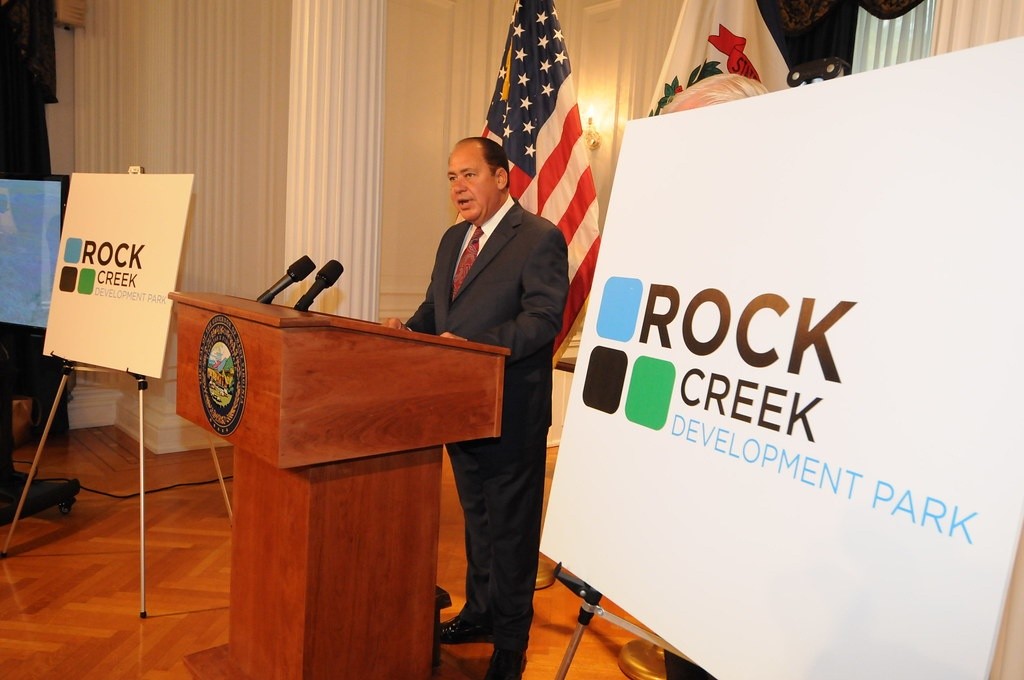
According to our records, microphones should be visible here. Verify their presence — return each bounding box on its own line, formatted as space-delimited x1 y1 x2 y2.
258 255 316 304
293 260 344 311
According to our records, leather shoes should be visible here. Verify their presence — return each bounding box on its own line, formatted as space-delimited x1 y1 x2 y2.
485 647 527 680
440 616 496 643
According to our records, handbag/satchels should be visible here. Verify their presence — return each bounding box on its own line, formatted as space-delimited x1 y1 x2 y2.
12 395 43 447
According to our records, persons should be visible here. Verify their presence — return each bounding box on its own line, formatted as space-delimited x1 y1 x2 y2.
378 136 570 680
661 73 769 679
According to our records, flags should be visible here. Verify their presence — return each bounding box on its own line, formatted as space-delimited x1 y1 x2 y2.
646 1 806 117
455 1 602 366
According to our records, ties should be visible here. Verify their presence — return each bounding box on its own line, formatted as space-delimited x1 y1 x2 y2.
450 226 484 302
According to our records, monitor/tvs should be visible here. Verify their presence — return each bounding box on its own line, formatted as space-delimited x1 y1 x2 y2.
0 170 70 336
